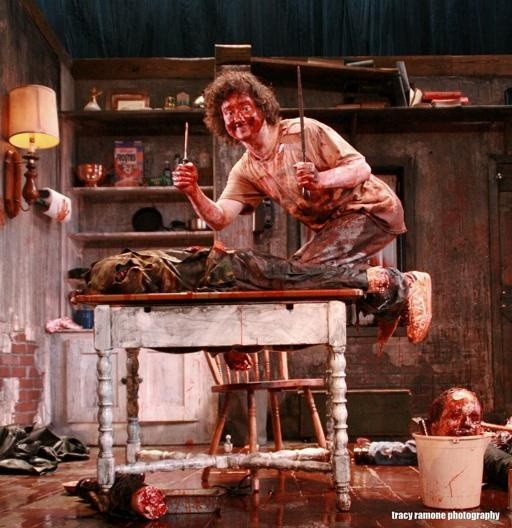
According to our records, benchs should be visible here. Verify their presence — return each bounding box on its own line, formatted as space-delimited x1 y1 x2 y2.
293 387 413 442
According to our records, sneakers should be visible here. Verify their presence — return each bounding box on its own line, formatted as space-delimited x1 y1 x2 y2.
400 269 433 345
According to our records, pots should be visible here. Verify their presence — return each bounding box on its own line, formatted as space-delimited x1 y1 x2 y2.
131 205 175 233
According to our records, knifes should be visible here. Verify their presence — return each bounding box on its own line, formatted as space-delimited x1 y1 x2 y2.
296 64 311 199
182 122 190 164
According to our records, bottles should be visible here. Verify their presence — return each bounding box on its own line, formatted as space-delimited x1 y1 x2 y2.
161 160 172 179
173 153 181 169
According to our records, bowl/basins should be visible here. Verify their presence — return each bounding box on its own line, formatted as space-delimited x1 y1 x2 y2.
189 216 211 230
62 479 81 496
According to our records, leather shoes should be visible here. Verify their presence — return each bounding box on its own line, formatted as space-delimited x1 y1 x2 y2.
353 436 370 465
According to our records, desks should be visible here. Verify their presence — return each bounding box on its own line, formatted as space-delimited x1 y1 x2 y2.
70 288 364 512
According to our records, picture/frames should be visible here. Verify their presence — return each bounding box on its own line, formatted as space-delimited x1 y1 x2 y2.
295 164 409 330
104 85 149 112
114 98 151 111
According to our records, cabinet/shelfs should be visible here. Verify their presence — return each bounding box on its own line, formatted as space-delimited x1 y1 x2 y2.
63 44 512 294
54 330 221 446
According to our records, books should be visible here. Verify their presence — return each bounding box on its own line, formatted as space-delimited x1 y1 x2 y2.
410 87 469 109
213 43 252 66
307 56 344 67
336 103 360 109
345 58 375 66
359 101 386 108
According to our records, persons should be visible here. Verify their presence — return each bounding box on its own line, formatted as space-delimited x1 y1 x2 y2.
427 387 483 436
68 248 434 374
172 68 410 358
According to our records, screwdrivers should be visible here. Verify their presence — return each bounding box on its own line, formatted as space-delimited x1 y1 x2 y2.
183 122 189 165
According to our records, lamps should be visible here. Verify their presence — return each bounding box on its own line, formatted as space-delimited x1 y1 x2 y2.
2 86 61 219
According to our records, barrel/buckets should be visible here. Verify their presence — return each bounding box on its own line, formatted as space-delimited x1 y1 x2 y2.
411 429 496 510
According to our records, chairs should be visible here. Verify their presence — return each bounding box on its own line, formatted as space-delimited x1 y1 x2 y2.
202 348 331 493
